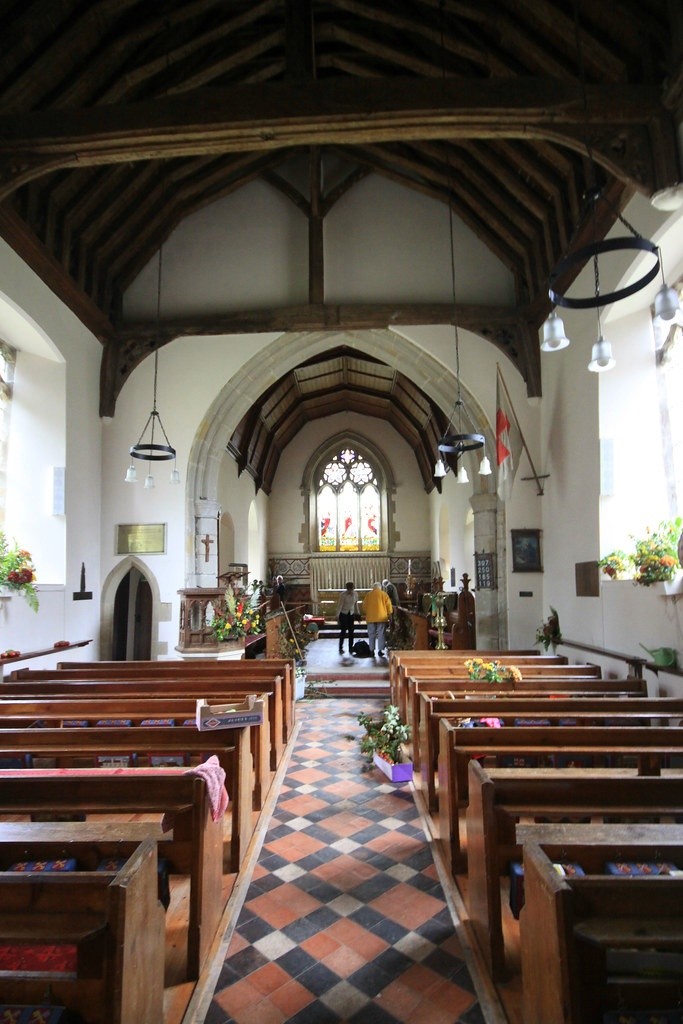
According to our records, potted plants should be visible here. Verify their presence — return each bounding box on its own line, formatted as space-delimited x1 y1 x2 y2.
353 700 416 783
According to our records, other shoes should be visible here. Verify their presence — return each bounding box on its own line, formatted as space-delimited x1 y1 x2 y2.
339 650 344 655
349 647 354 653
378 652 384 656
370 652 375 657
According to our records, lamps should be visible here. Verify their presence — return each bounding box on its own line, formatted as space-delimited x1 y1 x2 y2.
533 1 683 381
123 212 187 489
433 162 496 486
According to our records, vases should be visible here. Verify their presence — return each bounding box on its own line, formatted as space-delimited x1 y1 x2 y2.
227 636 247 647
602 567 621 583
292 675 308 702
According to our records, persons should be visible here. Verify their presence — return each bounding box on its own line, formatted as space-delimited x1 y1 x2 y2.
275 575 285 606
336 581 361 654
362 584 393 656
382 580 400 635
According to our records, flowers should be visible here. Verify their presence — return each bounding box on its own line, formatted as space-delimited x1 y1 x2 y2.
204 582 263 642
269 615 307 670
0 531 44 615
595 551 630 575
627 517 682 587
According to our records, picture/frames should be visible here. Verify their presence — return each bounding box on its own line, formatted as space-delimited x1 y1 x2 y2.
510 525 545 579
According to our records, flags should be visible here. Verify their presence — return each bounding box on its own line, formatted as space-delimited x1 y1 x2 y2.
495 368 525 501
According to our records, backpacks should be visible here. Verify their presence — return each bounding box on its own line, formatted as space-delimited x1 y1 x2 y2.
350 641 371 658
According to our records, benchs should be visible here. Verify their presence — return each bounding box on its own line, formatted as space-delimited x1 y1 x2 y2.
386 646 683 1024
0 654 301 1024
